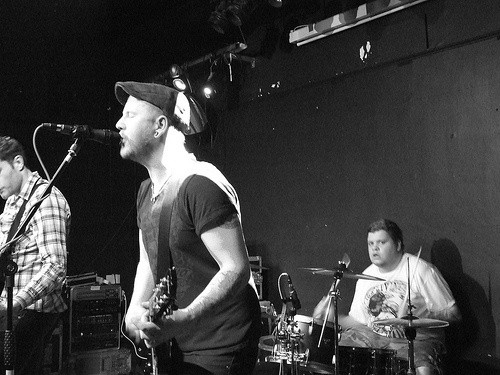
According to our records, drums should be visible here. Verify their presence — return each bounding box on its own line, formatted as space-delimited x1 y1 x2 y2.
269 313 343 365
334 345 398 375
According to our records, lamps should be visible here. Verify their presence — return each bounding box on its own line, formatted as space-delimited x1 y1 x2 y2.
289 0 430 46
168 0 282 99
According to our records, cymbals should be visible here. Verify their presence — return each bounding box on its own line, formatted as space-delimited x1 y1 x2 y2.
373 317 450 329
249 264 270 270
295 265 387 281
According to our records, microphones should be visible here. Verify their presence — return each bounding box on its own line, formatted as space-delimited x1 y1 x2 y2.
287 275 302 309
41 122 122 146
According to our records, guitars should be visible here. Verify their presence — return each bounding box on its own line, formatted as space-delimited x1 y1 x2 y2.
130 266 178 374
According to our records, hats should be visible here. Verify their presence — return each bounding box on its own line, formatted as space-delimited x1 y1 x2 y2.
115 81 191 136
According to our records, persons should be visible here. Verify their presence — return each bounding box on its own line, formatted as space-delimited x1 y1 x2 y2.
314 219 461 375
0 136 71 375
114 81 262 375
